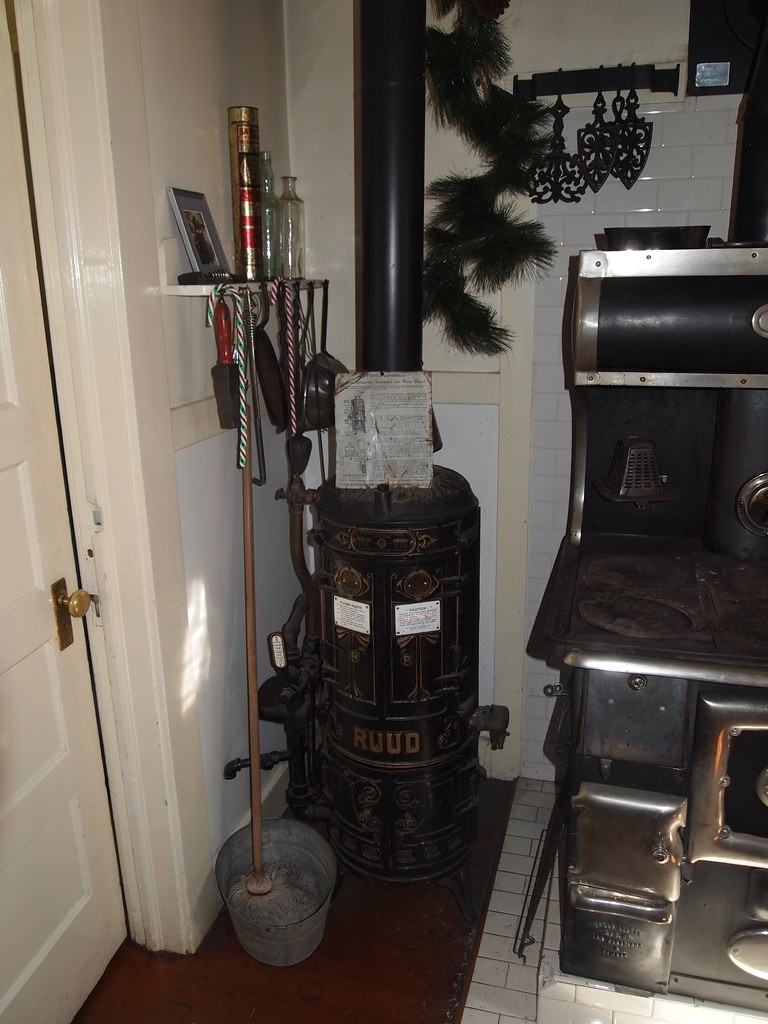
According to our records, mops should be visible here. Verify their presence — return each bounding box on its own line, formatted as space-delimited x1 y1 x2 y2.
226 400 321 929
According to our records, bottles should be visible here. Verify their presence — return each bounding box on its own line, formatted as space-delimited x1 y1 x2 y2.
279 175 305 279
259 151 283 279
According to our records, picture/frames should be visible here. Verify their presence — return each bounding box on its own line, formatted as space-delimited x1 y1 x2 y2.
167 186 232 280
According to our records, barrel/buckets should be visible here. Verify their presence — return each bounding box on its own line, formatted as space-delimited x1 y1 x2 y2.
215 818 345 966
